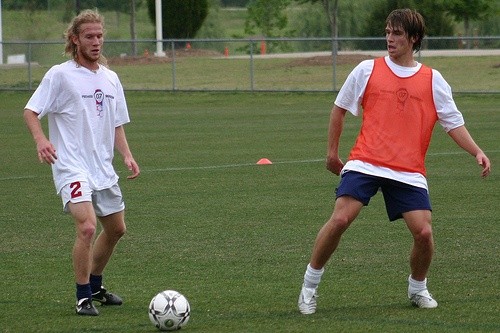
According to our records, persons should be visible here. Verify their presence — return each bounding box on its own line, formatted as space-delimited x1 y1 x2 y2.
298 6 492 316
23 7 142 315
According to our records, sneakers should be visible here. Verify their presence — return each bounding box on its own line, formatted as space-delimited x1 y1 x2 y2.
75 297 99 316
92 286 123 305
298 283 319 315
408 286 438 309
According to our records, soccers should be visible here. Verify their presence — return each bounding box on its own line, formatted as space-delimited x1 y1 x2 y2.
148 289 190 331
